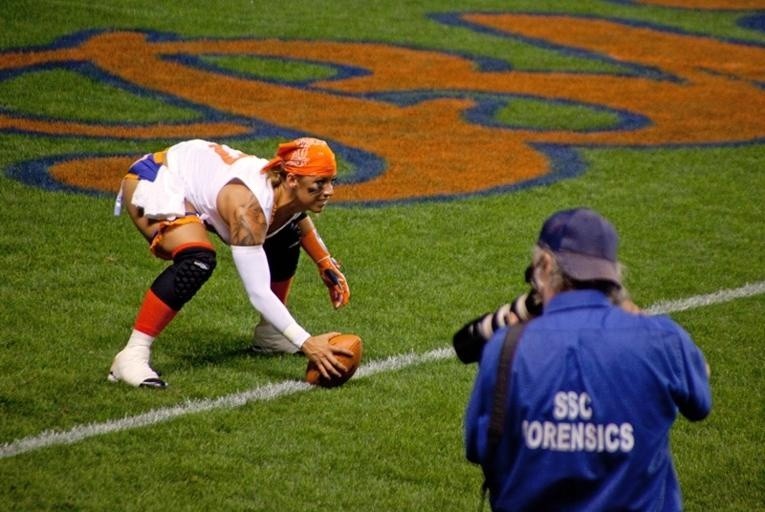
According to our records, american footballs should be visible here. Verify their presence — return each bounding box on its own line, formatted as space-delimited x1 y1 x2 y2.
306 335 362 387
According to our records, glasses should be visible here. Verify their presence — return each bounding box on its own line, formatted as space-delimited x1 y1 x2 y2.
522 253 548 286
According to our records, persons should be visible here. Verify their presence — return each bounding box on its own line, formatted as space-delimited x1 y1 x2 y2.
462 205 713 512
106 134 354 390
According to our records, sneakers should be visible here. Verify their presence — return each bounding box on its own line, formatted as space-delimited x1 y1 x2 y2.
106 343 169 390
250 321 306 357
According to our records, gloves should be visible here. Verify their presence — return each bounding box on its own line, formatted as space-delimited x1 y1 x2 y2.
318 258 350 309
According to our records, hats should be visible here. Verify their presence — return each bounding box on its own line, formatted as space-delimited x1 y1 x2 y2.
537 205 623 290
